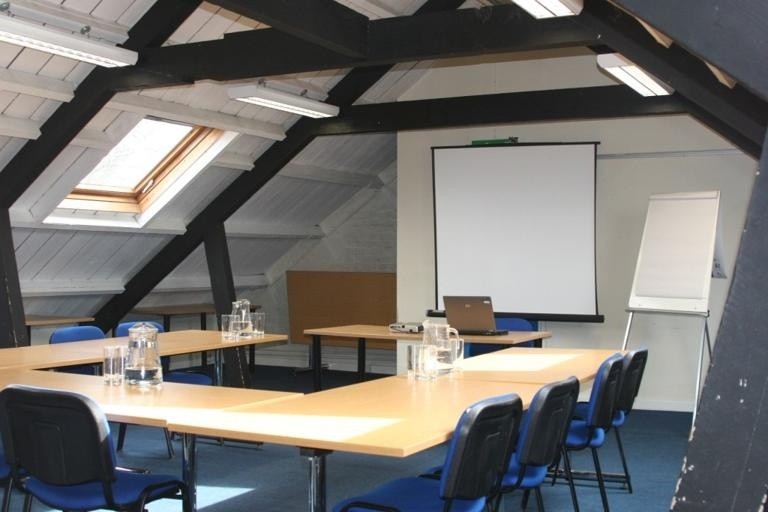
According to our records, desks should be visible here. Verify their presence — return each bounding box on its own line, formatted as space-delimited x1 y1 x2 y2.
0 329 289 445
302 324 552 391
0 368 305 512
131 303 262 374
24 314 95 346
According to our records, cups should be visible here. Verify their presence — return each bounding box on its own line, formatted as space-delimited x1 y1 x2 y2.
255 313 264 335
222 314 241 340
412 343 438 381
102 346 122 385
452 338 465 369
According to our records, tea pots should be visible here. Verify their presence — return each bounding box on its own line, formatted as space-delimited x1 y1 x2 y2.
423 320 460 375
124 320 164 394
231 298 253 339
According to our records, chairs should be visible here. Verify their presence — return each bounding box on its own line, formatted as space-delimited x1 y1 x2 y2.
421 375 579 512
330 393 523 510
565 352 625 512
115 322 212 458
49 326 107 375
1 383 187 511
552 346 648 494
462 316 539 358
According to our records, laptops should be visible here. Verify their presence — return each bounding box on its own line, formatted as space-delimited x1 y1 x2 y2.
443 296 508 336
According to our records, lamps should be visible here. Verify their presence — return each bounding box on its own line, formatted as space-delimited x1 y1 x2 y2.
226 78 341 122
1 3 139 70
595 53 673 100
512 0 585 21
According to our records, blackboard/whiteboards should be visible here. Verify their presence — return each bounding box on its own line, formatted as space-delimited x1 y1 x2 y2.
625 190 721 317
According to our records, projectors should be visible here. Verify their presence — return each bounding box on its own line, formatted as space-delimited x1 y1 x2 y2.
389 322 423 333
